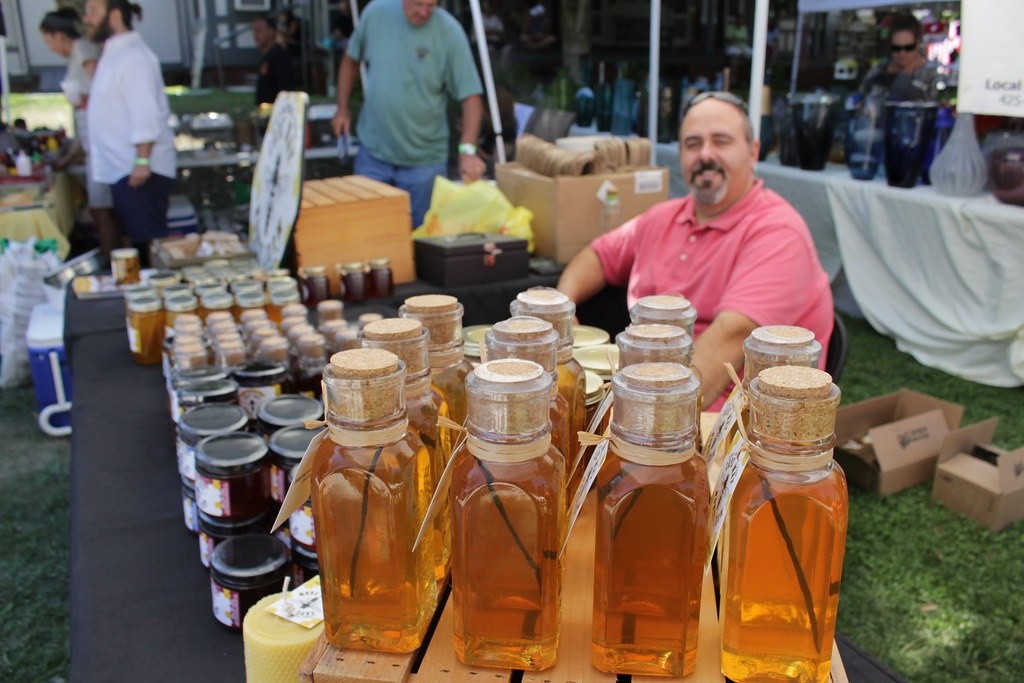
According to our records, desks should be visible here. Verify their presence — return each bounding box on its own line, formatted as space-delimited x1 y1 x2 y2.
63 266 912 683
0 103 349 262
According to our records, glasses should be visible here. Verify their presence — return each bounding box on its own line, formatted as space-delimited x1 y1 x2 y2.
890 37 918 51
684 91 748 116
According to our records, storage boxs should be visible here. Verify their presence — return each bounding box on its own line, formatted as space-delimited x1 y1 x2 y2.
167 188 199 238
28 302 73 437
833 389 966 500
932 416 1023 533
293 175 417 282
494 159 669 265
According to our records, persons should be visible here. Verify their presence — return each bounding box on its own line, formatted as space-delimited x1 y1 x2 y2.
557 92 834 413
332 0 488 229
84 0 184 267
40 7 102 140
858 12 939 101
253 17 293 135
276 0 354 54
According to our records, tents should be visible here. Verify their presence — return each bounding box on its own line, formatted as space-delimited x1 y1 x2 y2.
790 0 930 96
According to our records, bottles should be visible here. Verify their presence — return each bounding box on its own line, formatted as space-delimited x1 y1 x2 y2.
596 56 726 144
111 248 849 678
6 134 59 185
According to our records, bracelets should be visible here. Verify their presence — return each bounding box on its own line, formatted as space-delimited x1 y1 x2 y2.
135 158 150 166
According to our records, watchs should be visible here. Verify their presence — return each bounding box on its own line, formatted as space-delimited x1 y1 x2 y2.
458 143 477 155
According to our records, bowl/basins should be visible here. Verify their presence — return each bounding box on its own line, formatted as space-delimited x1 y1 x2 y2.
43 247 102 289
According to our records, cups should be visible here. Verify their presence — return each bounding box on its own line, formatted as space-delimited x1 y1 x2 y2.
884 98 939 188
790 92 841 171
